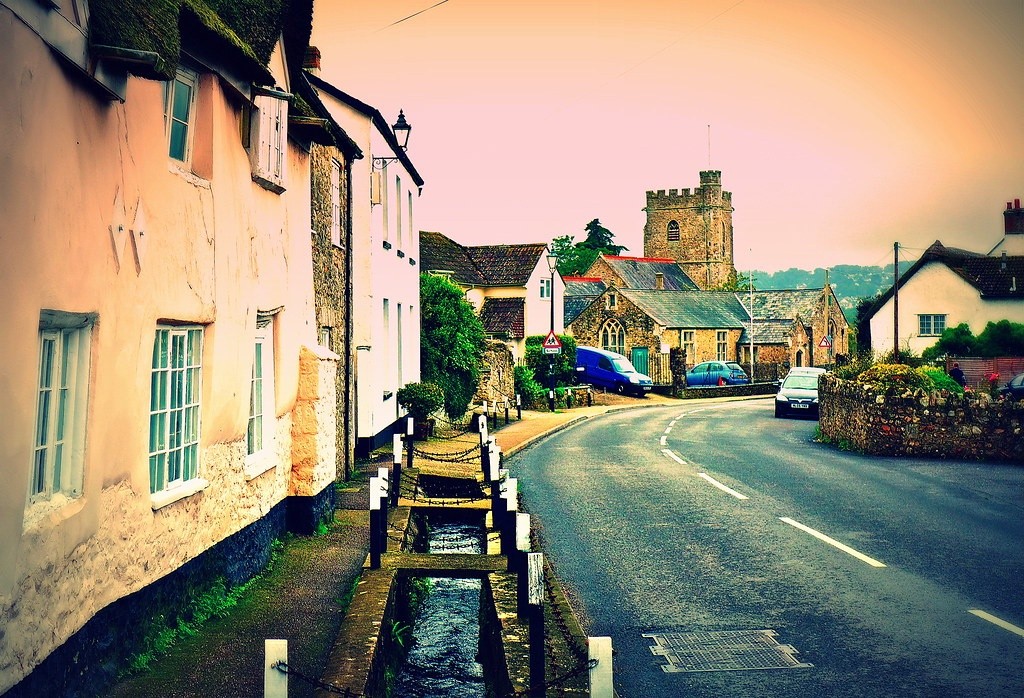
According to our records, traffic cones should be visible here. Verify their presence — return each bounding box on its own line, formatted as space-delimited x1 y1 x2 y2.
718 376 723 386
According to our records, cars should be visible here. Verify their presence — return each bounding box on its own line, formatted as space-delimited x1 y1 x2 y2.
686 360 750 386
775 366 827 418
997 373 1023 400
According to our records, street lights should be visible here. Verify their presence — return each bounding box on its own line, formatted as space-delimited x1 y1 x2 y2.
546 246 559 411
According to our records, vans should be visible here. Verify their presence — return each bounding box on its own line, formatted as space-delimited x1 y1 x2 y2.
576 346 652 395
